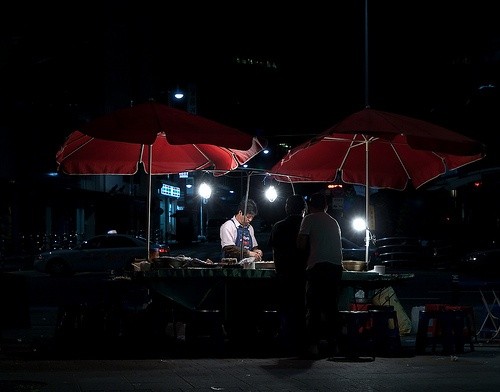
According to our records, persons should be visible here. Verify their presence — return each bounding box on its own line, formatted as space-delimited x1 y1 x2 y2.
269 195 310 356
220 199 263 262
297 200 341 359
168 208 198 248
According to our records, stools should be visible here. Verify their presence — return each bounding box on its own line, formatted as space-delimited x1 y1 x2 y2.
338 304 476 358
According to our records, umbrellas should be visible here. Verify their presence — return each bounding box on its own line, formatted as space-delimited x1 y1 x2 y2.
56 98 265 262
266 106 489 265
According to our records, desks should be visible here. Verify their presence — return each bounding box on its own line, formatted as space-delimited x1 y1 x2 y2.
121 267 416 359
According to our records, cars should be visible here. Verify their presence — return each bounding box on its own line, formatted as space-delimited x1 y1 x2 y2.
34 229 170 273
342 232 376 263
